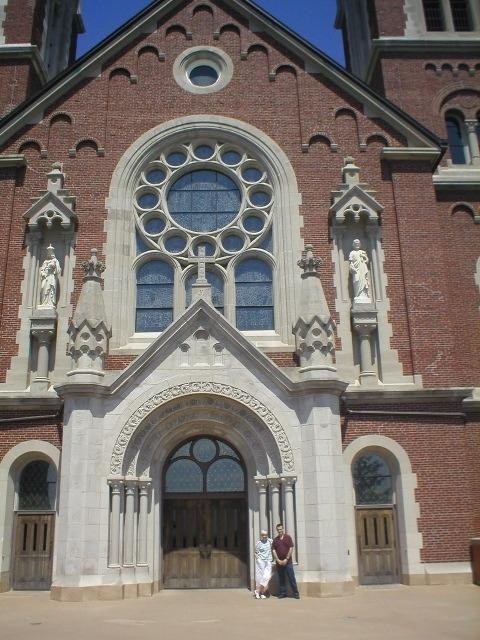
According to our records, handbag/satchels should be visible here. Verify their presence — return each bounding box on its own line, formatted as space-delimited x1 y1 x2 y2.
285 557 290 560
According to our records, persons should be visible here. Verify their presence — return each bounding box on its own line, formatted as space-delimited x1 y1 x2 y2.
349 239 370 297
254 530 276 599
272 524 299 599
41 247 62 305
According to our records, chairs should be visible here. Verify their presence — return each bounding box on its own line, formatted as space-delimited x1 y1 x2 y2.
253 589 271 599
279 593 288 598
293 592 300 599
